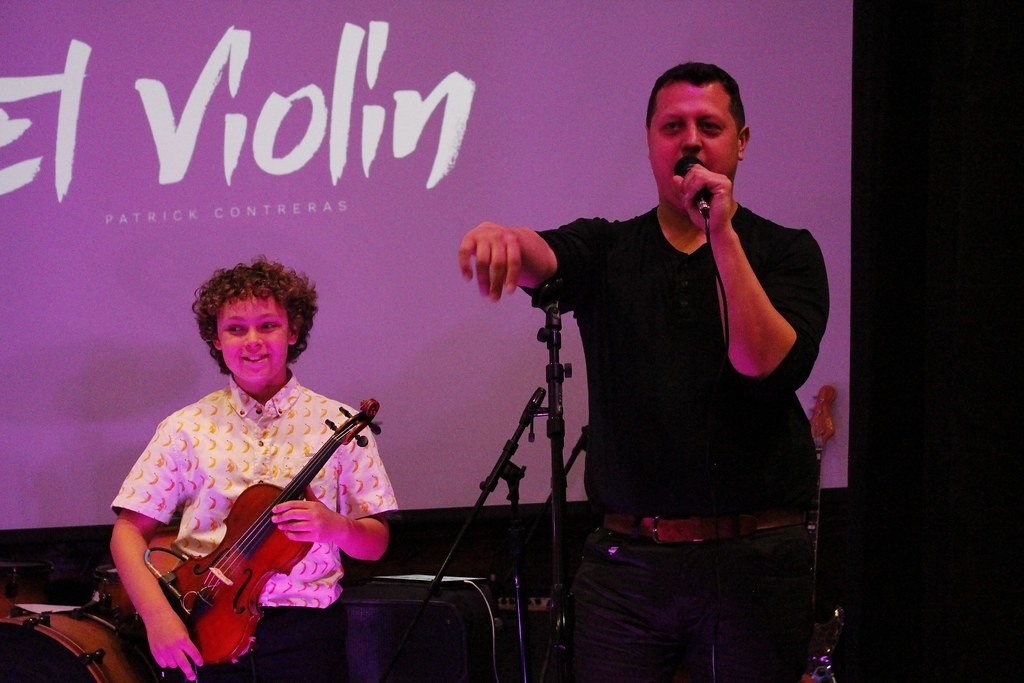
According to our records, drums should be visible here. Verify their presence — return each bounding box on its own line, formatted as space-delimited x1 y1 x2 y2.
0 603 160 683
0 560 55 617
91 560 143 625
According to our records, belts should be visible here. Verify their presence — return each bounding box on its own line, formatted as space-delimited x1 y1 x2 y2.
604 507 807 545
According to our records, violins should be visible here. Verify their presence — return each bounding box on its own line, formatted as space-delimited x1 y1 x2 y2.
142 397 383 664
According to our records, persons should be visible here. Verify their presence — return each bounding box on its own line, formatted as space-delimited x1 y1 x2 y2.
109 254 399 683
459 61 830 683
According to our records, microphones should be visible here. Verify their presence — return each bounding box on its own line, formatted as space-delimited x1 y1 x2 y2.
675 155 709 219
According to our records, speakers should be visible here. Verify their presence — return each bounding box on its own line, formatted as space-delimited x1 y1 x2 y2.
344 583 495 683
496 596 575 683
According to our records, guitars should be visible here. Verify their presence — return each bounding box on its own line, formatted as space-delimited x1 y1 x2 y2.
797 383 845 683
